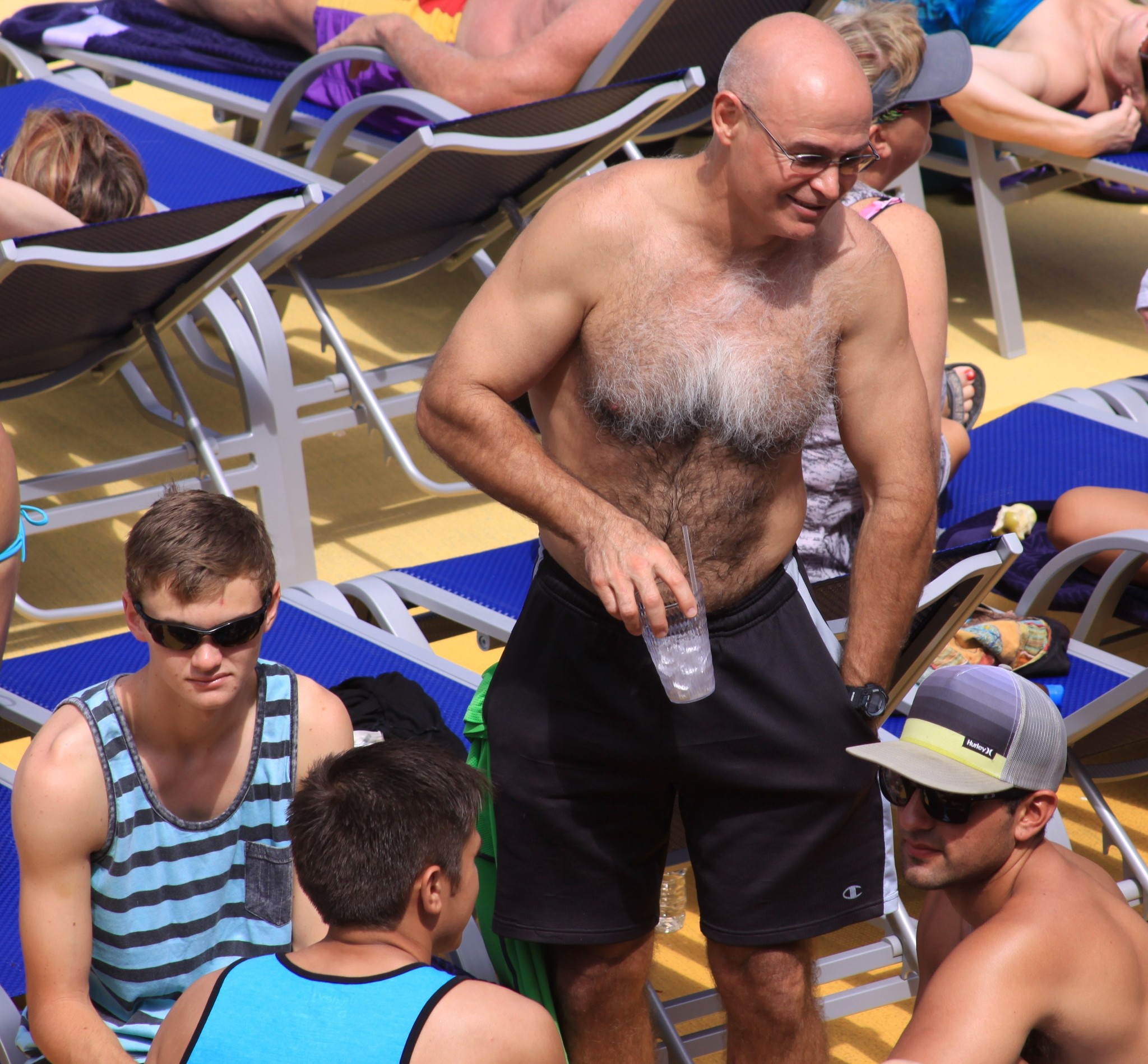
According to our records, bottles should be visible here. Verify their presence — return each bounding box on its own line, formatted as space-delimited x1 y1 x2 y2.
654 868 687 935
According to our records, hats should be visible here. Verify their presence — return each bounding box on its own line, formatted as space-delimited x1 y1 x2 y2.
870 29 973 119
845 664 1067 795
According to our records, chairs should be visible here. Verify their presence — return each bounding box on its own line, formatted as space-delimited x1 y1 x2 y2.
0 0 1148 1064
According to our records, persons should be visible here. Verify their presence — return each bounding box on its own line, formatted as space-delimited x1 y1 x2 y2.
0 0 1148 1064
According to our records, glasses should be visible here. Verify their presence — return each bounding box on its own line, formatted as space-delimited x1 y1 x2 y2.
879 764 1033 824
132 590 273 650
0 146 12 176
875 98 931 123
739 100 880 175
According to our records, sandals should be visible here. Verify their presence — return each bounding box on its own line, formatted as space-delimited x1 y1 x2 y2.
941 363 986 433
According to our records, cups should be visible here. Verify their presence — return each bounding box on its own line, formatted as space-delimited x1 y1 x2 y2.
635 577 716 705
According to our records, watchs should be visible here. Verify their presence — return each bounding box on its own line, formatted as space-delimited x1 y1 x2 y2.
845 683 889 719
